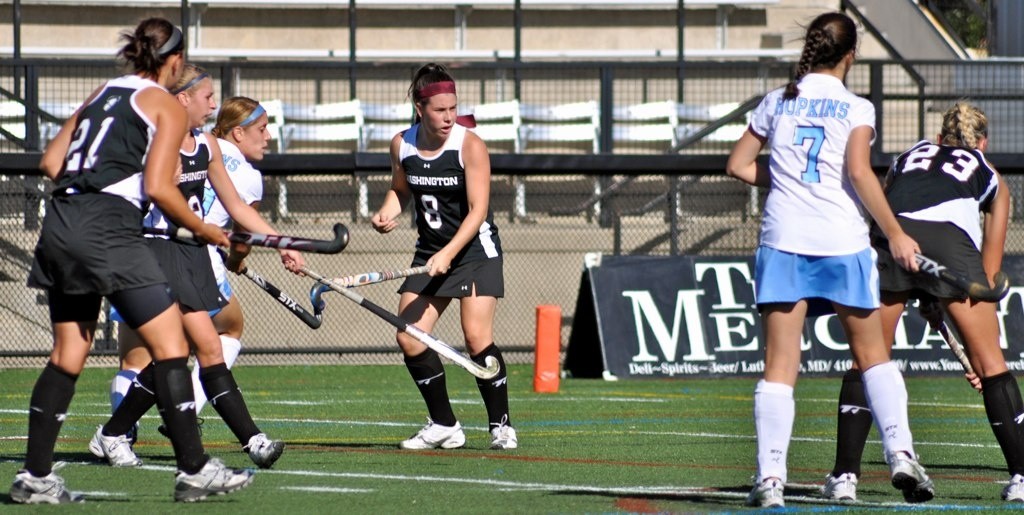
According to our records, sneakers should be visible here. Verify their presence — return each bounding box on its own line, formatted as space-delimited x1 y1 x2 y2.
489 413 518 449
159 418 205 438
888 451 935 503
819 472 858 500
400 417 464 449
243 433 284 469
174 457 254 501
88 423 137 466
746 478 785 508
10 461 84 504
1002 474 1024 501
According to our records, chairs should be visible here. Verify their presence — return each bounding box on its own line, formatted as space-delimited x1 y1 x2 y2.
0 99 763 224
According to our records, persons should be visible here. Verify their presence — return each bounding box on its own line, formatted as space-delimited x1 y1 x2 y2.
371 61 518 451
725 11 935 508
86 63 309 469
823 104 1024 502
7 18 254 506
109 96 272 447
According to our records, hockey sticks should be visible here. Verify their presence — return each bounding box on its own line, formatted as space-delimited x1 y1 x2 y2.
920 304 982 383
220 251 322 330
309 267 443 311
133 221 351 256
289 261 500 379
867 231 1010 303
